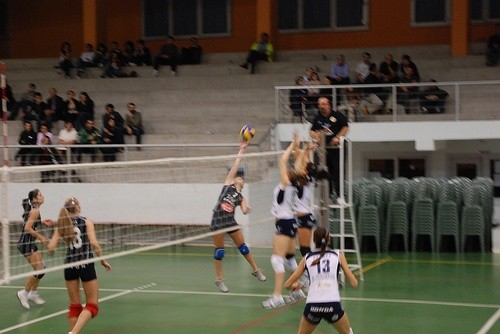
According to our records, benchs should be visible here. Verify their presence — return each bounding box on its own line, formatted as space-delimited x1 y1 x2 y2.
0 43 500 181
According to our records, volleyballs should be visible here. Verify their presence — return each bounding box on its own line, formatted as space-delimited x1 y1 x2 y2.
239 124 257 143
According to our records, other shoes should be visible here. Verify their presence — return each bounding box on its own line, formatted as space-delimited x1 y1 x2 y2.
319 197 335 204
336 197 346 205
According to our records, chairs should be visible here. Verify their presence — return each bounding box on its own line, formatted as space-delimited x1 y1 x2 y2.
326 175 495 255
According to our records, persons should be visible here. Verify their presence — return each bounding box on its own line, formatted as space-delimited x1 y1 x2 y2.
16 189 49 310
54 35 204 79
283 226 358 334
309 96 348 206
210 141 267 292
0 79 144 183
262 131 330 310
240 32 274 75
47 197 112 334
289 52 452 115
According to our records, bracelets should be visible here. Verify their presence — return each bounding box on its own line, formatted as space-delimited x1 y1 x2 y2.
335 135 340 140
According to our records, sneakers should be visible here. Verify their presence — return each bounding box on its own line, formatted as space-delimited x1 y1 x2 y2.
28 291 46 305
215 279 228 292
286 289 307 305
252 268 267 281
17 289 30 309
299 277 310 288
261 296 287 309
